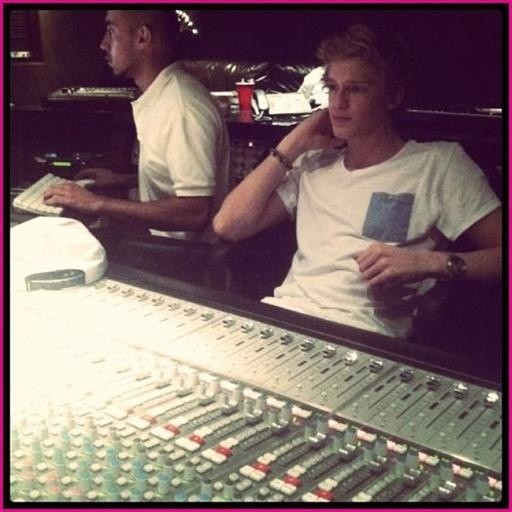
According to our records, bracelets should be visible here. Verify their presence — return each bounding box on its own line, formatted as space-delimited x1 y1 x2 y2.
270 148 294 171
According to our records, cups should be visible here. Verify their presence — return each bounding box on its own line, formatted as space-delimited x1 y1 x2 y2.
235 81 255 111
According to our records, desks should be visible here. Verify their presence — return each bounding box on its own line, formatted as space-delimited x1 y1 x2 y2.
7 94 316 299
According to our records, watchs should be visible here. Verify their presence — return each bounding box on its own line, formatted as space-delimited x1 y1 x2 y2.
445 251 469 285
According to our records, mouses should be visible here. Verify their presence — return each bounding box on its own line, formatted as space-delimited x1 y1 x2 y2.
76 178 96 188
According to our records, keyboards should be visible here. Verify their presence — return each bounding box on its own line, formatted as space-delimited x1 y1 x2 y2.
12 171 85 217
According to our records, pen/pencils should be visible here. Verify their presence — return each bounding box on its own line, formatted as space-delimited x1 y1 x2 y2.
51 161 74 167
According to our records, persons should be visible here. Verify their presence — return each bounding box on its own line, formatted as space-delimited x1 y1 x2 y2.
212 25 502 340
43 8 232 293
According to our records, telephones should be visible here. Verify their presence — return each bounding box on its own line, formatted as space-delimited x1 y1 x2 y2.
250 89 313 116
